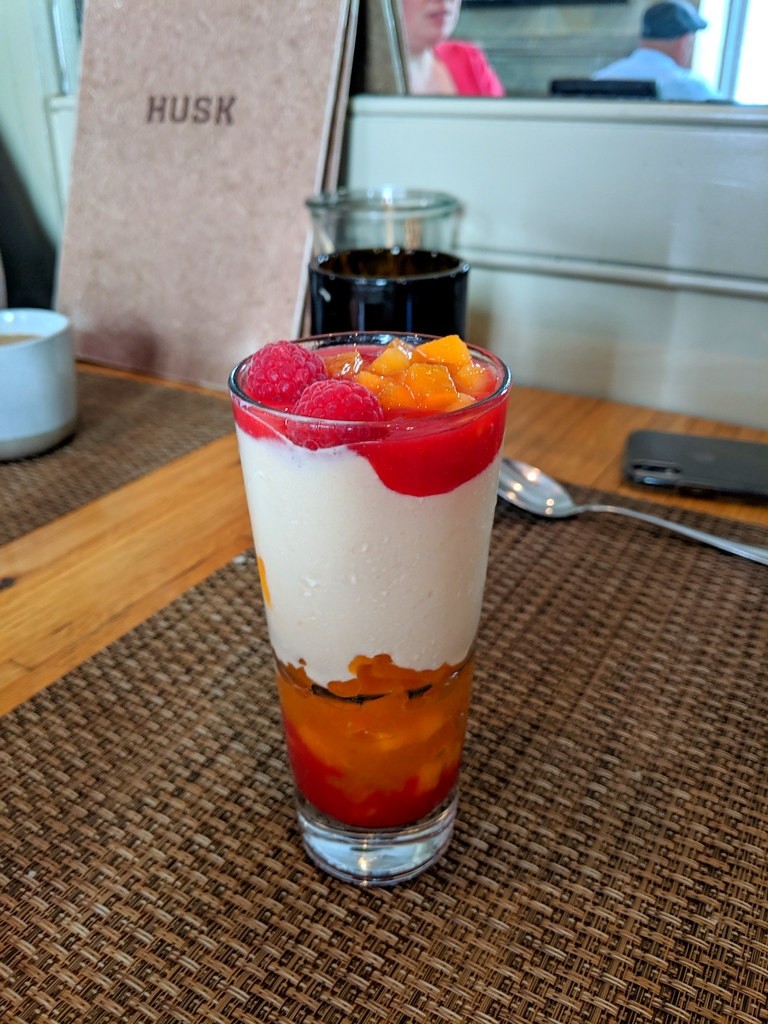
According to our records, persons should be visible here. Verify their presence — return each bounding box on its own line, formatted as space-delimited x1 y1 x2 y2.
592 0 724 102
398 0 504 97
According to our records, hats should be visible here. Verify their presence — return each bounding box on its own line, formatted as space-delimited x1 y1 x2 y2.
641 2 708 38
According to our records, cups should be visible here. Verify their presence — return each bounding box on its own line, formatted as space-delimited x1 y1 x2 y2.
226 331 515 887
305 185 473 343
0 309 81 460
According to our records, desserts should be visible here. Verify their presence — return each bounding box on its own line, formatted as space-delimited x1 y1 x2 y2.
231 335 510 830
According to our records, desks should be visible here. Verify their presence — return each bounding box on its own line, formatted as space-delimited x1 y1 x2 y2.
0 359 768 1024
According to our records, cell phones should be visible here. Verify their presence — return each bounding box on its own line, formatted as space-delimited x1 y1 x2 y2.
620 428 768 505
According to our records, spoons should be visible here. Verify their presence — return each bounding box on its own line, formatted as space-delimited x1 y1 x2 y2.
497 456 767 566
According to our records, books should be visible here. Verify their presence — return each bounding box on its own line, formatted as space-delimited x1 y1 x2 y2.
49 0 357 394
